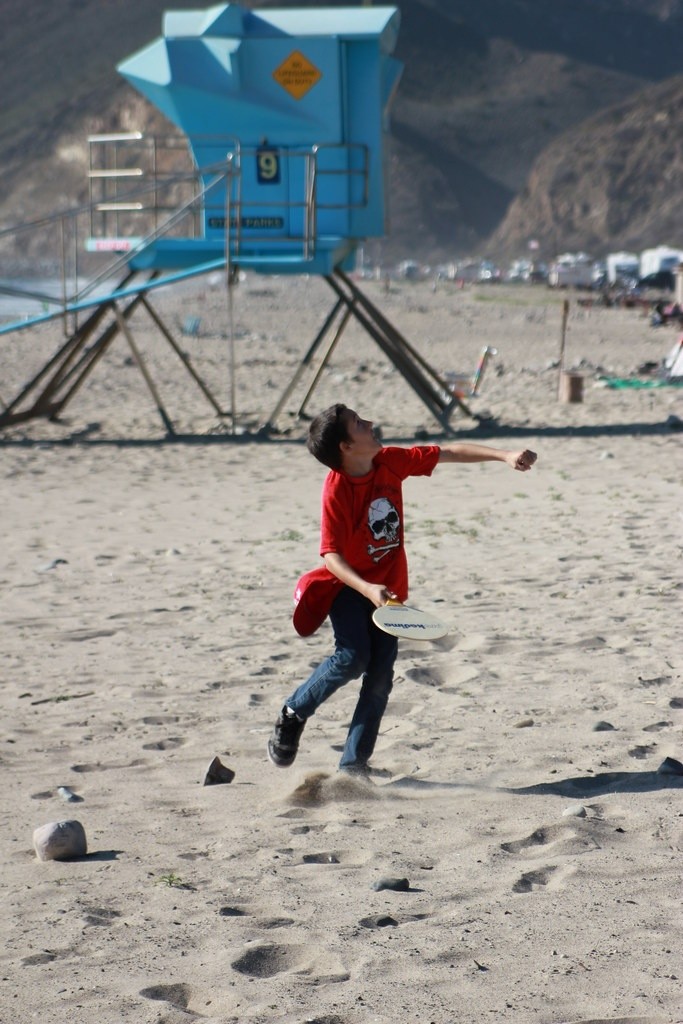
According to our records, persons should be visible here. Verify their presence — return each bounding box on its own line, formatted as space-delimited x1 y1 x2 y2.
269 404 538 784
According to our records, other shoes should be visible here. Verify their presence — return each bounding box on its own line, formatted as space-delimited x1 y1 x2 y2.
338 769 376 786
268 705 307 769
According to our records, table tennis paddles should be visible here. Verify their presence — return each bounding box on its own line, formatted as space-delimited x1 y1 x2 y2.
373 597 449 641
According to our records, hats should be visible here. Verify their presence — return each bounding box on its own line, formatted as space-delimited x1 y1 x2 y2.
292 567 344 638
